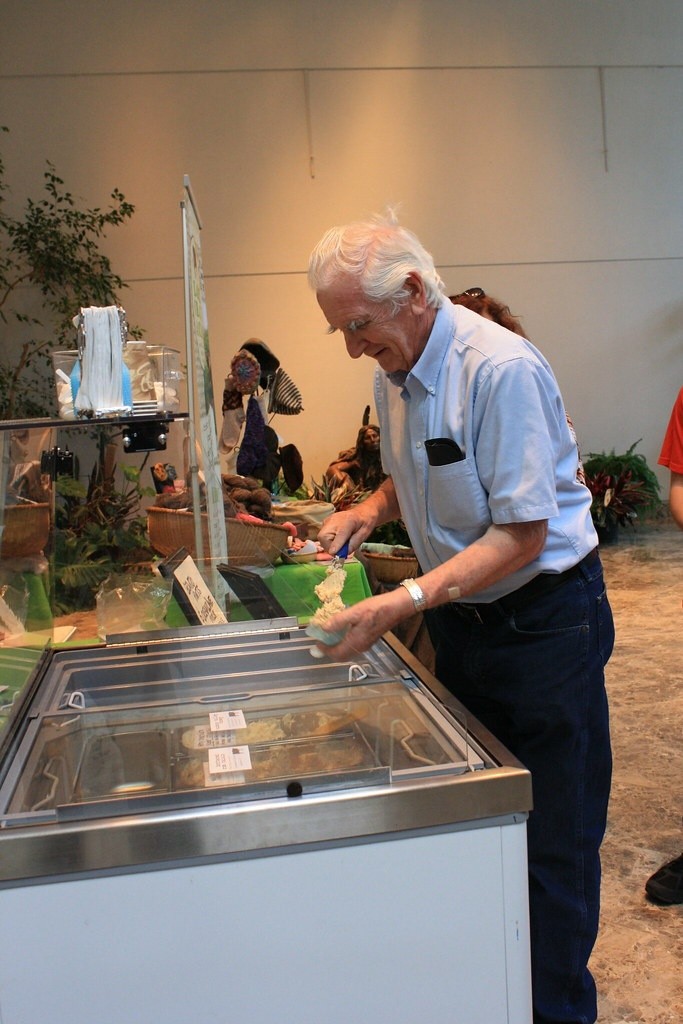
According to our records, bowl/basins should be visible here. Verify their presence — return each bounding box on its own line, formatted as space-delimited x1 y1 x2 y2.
305 622 349 644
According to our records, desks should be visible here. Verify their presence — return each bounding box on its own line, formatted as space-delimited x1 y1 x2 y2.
142 556 374 630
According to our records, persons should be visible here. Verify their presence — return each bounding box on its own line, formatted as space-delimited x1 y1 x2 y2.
310 207 618 1024
448 286 586 486
644 385 683 903
325 424 388 511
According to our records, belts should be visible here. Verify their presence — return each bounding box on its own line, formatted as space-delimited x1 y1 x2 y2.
444 544 599 624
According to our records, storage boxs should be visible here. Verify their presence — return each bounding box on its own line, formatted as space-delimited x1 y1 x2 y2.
48 344 181 418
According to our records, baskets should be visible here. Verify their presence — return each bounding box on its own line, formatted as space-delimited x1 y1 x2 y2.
146 505 291 567
361 550 418 583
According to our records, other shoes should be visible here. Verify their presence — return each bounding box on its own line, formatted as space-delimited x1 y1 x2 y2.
646 855 683 904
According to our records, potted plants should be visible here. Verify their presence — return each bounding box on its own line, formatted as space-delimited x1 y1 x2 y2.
581 466 657 536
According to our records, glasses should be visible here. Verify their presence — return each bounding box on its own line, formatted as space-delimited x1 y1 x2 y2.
448 286 484 302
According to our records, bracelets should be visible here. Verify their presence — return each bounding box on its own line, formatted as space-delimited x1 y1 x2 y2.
399 577 427 612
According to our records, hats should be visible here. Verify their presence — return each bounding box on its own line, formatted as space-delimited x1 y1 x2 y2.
267 369 302 416
230 350 261 394
238 338 280 389
279 444 303 494
